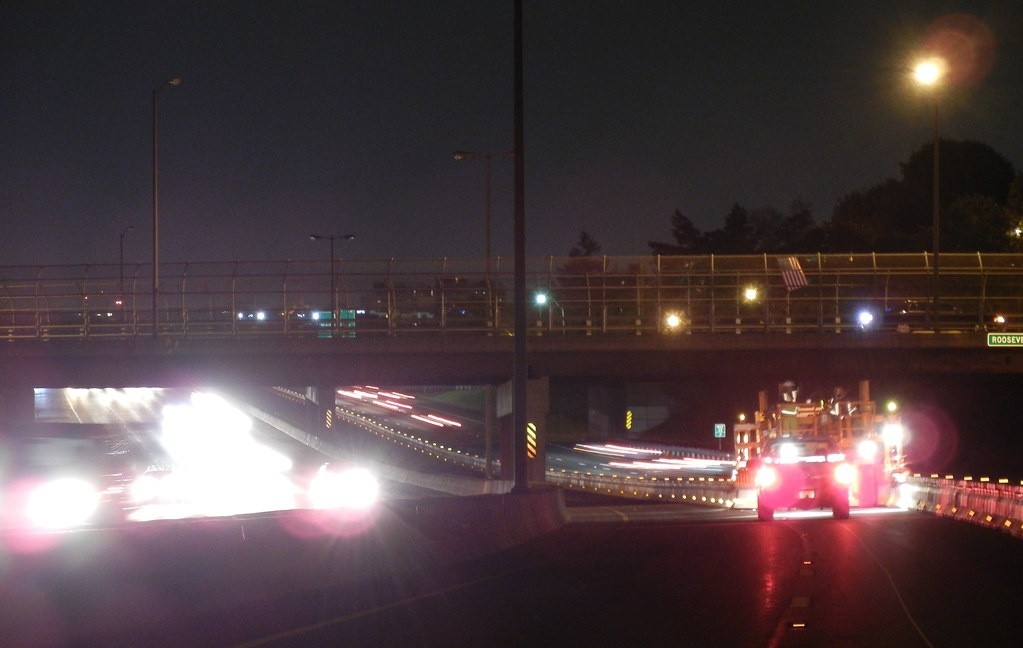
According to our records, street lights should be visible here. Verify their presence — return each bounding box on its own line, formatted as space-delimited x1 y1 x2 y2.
119 225 135 323
911 55 947 330
152 78 185 330
308 234 355 332
452 148 517 327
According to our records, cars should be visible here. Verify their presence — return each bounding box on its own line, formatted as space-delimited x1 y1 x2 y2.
756 435 853 521
